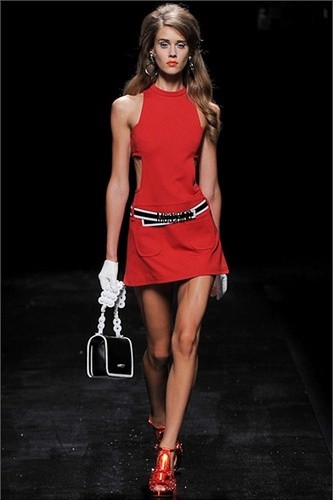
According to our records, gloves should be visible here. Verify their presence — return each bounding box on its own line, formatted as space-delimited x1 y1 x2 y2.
209 273 226 300
97 260 118 292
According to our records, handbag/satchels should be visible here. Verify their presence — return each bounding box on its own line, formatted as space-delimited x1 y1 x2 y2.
85 281 133 379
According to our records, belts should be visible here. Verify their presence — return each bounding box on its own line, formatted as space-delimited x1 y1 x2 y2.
130 199 208 227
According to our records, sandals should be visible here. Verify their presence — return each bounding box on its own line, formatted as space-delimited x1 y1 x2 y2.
149 442 178 496
147 414 182 457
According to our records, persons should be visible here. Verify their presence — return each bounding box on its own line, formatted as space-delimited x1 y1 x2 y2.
97 2 227 497
